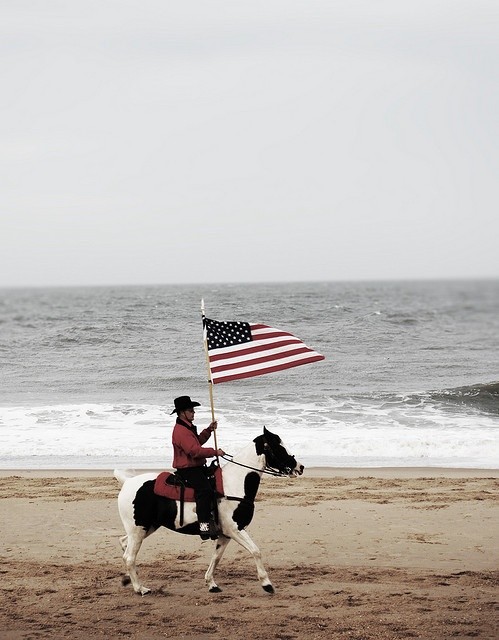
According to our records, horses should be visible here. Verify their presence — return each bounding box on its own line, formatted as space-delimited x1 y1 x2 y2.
113 426 305 596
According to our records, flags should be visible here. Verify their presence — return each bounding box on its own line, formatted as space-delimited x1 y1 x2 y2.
202 315 325 384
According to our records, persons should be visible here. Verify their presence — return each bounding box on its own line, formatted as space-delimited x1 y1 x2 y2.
170 396 224 540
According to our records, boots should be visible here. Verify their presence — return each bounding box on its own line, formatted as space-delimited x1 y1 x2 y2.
199 522 222 541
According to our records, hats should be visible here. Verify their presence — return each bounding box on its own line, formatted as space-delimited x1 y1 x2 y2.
170 395 202 423
